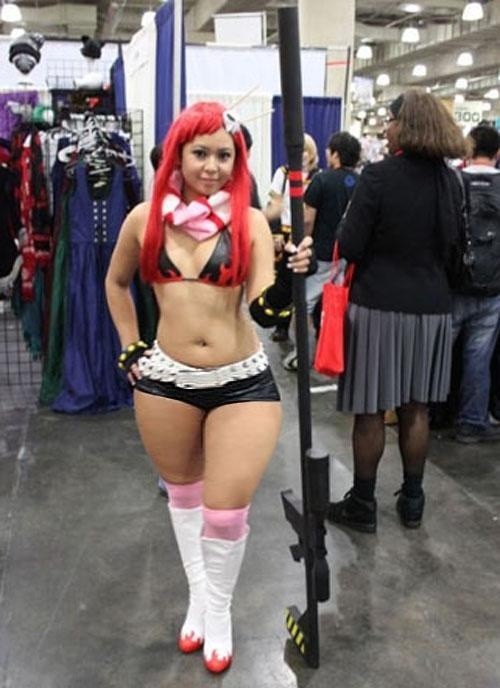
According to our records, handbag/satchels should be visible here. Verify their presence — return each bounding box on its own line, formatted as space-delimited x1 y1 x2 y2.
450 208 470 297
313 262 354 377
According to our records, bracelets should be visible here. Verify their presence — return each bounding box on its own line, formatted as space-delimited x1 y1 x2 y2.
118 338 149 376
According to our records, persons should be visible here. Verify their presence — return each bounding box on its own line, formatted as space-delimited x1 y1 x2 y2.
322 90 473 536
1 136 23 300
105 98 317 679
238 125 360 371
458 126 499 445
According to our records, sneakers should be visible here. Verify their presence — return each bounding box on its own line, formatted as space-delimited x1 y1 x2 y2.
269 326 298 371
396 492 425 528
455 425 500 444
326 495 377 532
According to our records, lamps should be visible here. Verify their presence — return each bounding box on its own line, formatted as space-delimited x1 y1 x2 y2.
356 1 499 111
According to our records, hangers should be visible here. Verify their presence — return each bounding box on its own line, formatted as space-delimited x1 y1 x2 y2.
59 117 135 168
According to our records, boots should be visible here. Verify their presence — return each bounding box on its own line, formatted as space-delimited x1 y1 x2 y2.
200 523 250 673
167 502 205 654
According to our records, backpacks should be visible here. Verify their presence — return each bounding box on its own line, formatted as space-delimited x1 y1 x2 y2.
468 176 500 298
